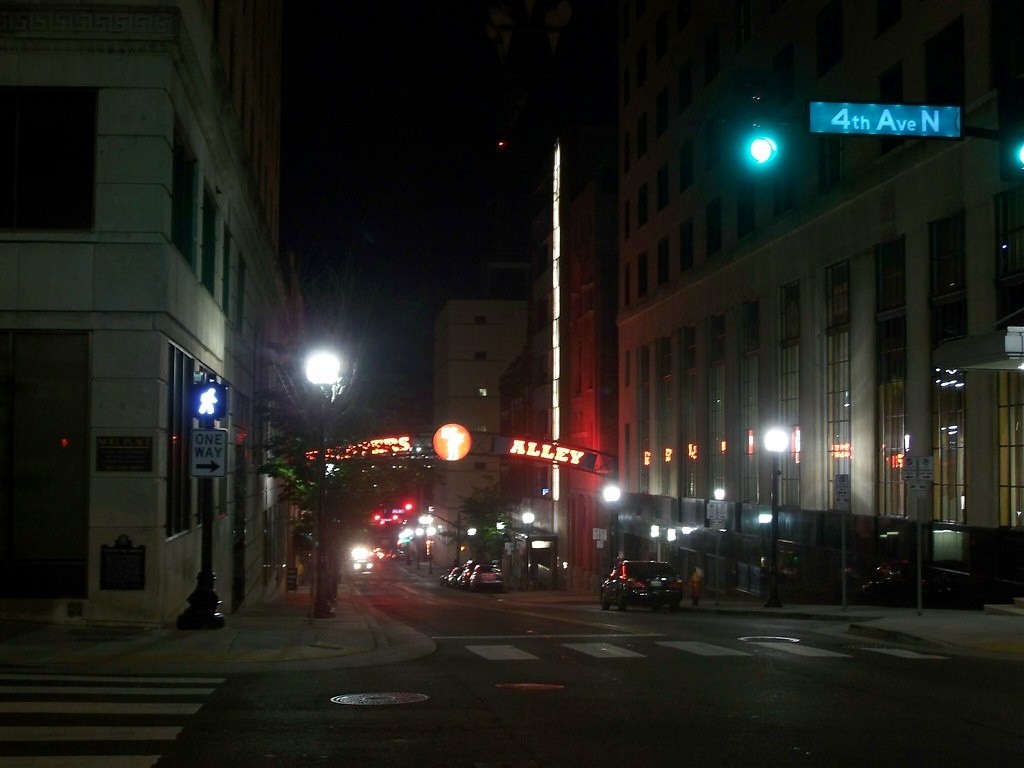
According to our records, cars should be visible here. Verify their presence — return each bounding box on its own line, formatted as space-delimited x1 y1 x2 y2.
447 557 506 592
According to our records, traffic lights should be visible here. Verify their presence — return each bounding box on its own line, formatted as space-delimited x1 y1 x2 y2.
188 379 227 423
997 73 1024 183
743 70 780 171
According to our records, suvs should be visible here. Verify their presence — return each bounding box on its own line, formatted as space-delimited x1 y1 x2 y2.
599 558 685 612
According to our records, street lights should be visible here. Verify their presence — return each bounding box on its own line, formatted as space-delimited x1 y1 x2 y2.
521 512 536 579
763 429 800 608
415 528 424 569
603 485 621 571
426 527 437 574
302 330 351 620
